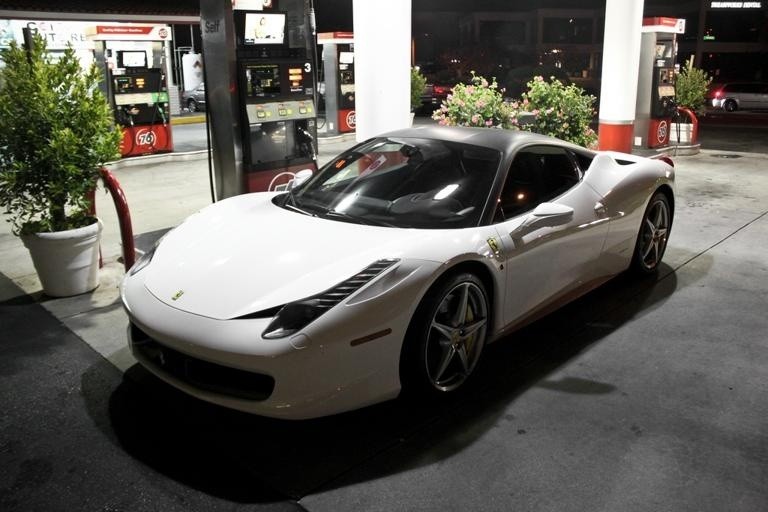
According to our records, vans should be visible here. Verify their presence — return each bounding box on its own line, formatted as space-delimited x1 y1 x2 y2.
712 82 768 112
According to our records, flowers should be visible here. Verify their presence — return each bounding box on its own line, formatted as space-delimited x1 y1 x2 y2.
430 70 599 150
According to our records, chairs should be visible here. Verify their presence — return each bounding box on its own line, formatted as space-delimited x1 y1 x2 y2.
358 152 558 216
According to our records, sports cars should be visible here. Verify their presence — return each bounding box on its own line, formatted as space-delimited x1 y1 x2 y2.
120 127 674 421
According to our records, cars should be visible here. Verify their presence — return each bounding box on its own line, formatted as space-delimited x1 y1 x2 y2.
415 62 472 105
317 69 355 110
181 82 206 112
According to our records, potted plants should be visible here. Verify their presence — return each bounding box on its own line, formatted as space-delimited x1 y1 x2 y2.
410 65 427 127
1 28 125 298
670 54 713 144
